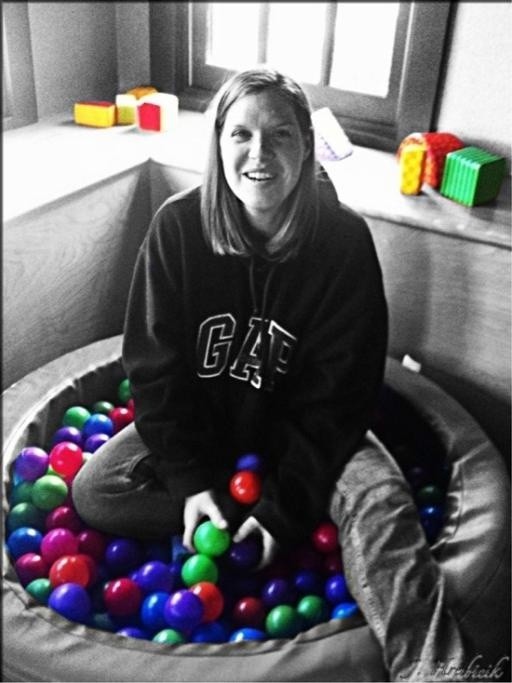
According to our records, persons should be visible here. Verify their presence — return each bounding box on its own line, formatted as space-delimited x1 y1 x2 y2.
7 378 445 648
70 69 466 683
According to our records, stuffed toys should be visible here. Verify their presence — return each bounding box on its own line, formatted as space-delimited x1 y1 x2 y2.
74 87 179 134
395 131 506 207
6 379 445 647
